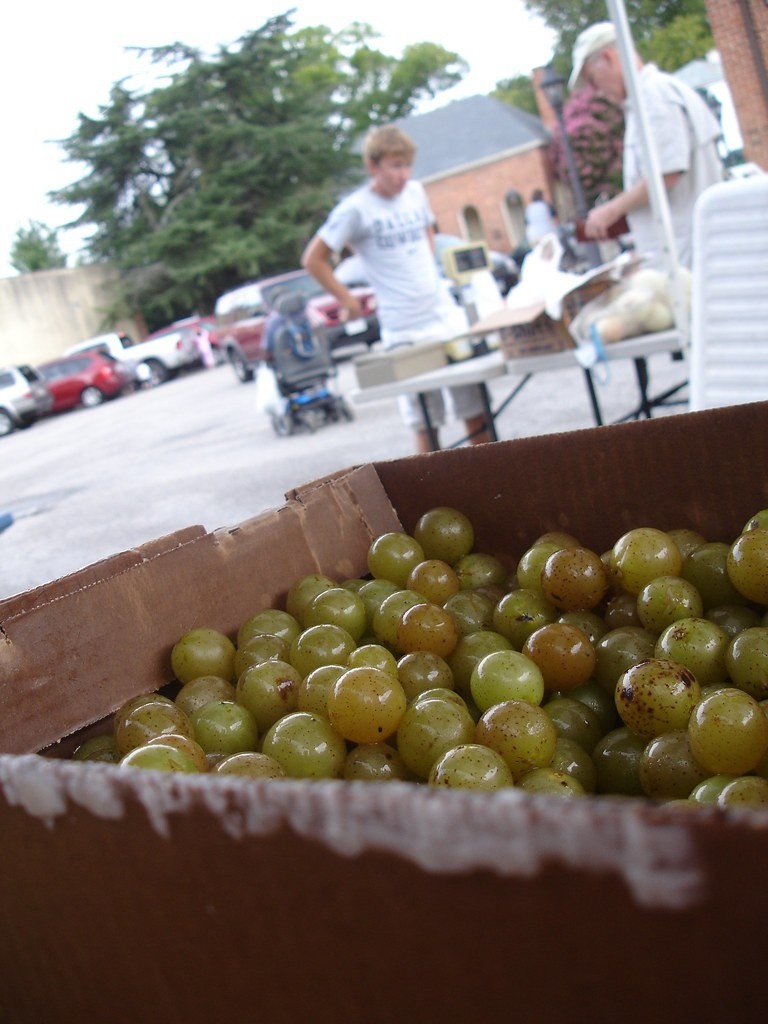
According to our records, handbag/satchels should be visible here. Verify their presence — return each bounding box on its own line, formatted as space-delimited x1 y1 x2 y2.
569 268 673 373
506 234 574 320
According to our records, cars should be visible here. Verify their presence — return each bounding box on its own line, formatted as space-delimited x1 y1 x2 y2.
304 233 521 357
29 347 132 412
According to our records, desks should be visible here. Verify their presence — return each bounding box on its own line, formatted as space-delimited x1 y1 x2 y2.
348 328 688 451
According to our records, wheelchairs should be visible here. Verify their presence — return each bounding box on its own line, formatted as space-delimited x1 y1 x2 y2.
264 294 355 436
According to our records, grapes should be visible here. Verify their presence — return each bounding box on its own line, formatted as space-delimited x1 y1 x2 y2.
69 510 768 812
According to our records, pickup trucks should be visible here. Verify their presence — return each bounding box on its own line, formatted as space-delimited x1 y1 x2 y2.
60 330 200 389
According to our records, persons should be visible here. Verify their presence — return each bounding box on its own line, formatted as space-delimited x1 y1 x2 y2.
262 288 308 367
300 129 496 455
567 22 725 275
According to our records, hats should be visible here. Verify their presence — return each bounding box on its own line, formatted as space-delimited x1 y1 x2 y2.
568 22 617 90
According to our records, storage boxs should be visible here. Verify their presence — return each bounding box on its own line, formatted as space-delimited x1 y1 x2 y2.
0 400 768 1024
353 338 450 389
466 261 620 359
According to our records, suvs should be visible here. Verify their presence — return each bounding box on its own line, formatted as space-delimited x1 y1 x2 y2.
211 267 378 384
0 360 54 438
